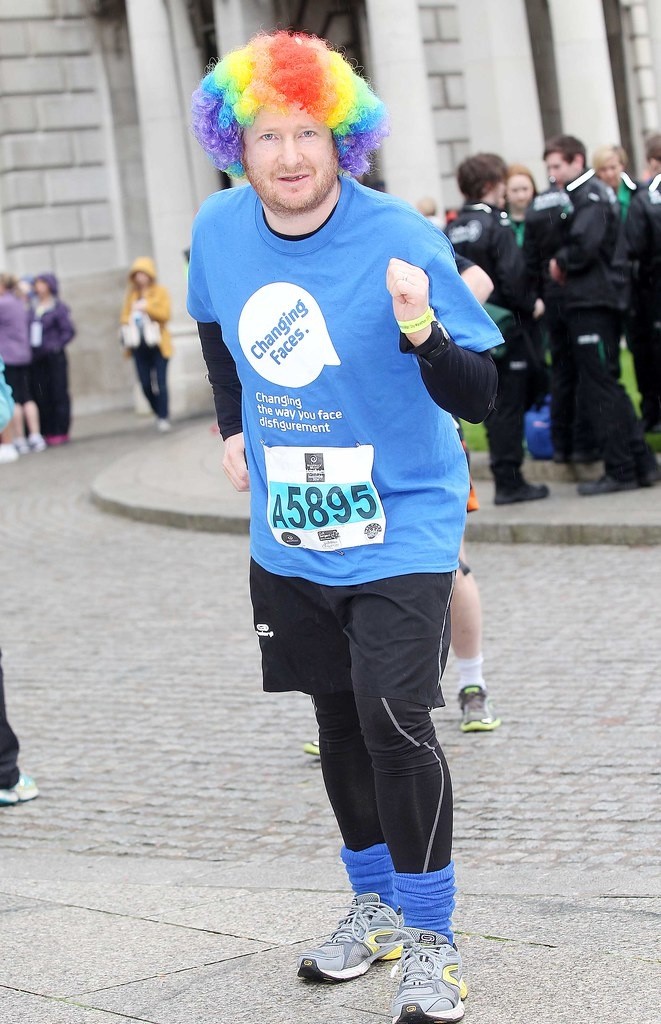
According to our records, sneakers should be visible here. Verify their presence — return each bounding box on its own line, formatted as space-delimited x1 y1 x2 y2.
296 893 405 983
0 773 39 806
303 738 320 756
373 922 470 1023
457 684 500 733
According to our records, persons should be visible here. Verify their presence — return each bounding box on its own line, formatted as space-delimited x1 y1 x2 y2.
302 411 503 754
0 641 41 806
0 271 75 462
448 131 661 510
186 26 506 1023
116 253 175 435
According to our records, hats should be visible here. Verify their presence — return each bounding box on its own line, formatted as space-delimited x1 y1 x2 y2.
193 29 389 177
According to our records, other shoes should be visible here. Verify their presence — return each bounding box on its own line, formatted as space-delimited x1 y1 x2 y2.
576 464 661 494
0 444 18 463
157 420 170 433
493 481 548 504
16 436 45 455
44 435 68 446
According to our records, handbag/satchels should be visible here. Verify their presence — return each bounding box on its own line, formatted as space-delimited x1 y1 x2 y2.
121 301 161 350
523 396 556 462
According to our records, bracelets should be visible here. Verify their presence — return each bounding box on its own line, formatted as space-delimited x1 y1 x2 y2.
393 307 436 335
